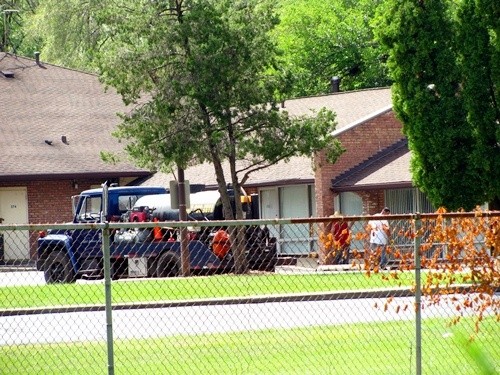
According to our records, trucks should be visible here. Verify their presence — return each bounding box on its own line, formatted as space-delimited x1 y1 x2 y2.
40 185 278 278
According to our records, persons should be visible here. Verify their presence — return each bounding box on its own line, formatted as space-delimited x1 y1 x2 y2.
330 215 350 264
369 207 391 270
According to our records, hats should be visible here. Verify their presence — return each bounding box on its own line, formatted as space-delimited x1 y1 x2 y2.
329 211 344 217
